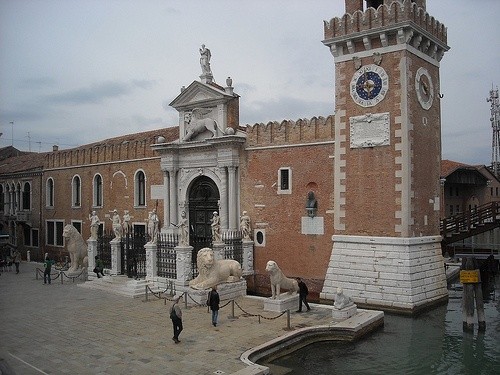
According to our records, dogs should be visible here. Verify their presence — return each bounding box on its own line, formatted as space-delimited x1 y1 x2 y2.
265 260 299 300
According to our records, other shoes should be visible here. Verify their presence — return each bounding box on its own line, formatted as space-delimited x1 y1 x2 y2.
213 321 216 327
171 338 178 343
175 341 181 343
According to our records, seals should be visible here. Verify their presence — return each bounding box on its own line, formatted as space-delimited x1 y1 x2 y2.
333 287 353 309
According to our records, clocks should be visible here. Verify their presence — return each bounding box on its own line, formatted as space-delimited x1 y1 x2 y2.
348 64 389 106
414 66 435 110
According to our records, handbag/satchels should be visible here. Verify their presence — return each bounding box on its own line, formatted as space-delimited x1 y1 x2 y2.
206 300 210 307
170 311 178 319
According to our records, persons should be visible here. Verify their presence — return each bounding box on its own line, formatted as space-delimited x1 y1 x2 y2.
295 277 310 312
14 248 21 274
169 305 183 343
43 255 55 285
0 253 13 272
93 256 105 278
207 286 220 327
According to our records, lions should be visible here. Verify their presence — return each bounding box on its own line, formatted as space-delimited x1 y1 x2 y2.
62 224 88 271
189 247 243 288
